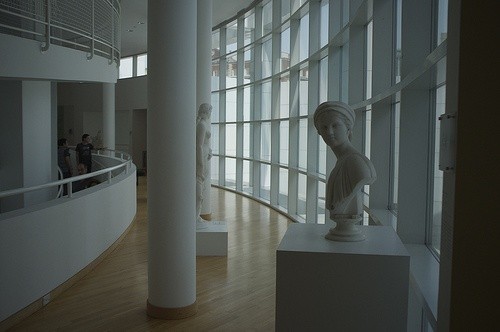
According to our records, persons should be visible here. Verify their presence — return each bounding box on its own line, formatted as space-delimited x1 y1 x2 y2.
314 100 379 219
76 133 96 188
58 136 75 197
197 102 213 224
69 162 98 194
